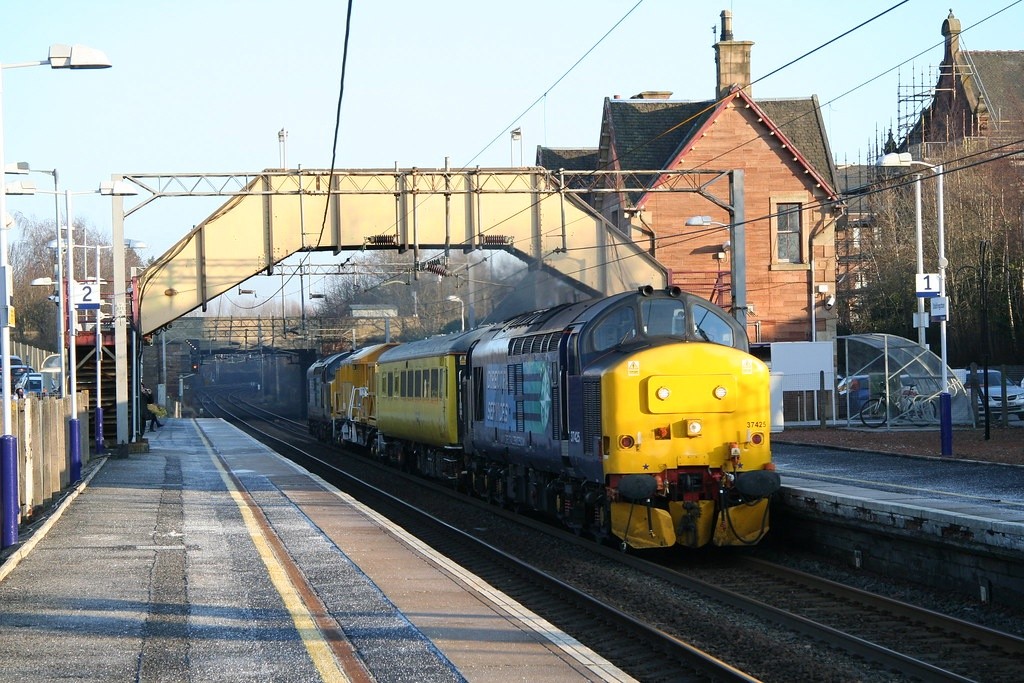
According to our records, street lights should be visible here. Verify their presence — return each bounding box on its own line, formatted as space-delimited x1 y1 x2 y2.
447 296 467 331
6 181 82 484
873 152 955 454
46 239 108 452
3 168 64 396
2 42 113 546
60 225 89 328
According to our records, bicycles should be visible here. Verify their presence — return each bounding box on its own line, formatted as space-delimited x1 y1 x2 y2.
860 381 938 429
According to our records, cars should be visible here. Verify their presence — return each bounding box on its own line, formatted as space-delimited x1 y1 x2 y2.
949 369 1023 419
10 364 36 393
0 354 22 385
15 374 41 399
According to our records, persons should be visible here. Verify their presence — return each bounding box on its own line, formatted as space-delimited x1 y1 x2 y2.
13 387 26 400
140 383 165 438
42 387 49 396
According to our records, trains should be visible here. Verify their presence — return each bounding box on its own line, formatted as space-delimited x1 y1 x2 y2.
306 285 780 553
